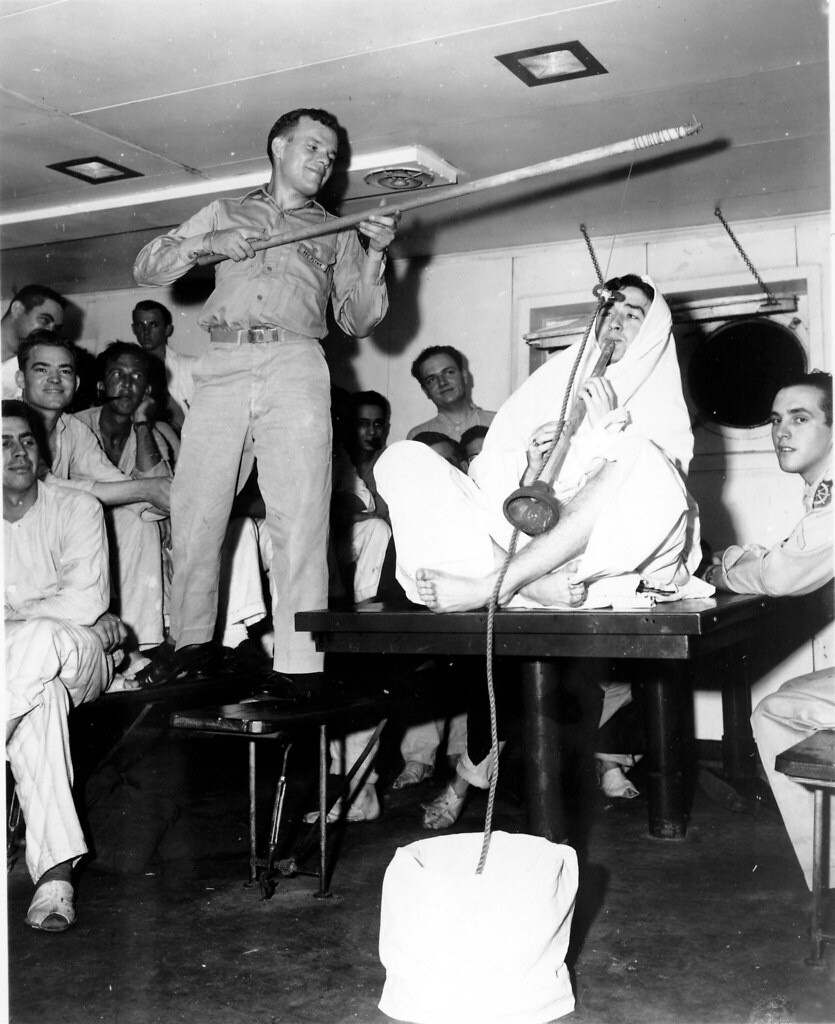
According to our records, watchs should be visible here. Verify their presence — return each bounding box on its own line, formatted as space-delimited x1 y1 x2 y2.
705 564 722 583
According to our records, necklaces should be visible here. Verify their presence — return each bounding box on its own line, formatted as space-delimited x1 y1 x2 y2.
439 408 479 432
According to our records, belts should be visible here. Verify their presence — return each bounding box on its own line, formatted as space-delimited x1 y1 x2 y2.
210 329 312 341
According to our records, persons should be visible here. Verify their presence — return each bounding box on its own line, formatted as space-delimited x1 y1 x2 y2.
372 272 696 615
130 108 399 680
1 281 835 941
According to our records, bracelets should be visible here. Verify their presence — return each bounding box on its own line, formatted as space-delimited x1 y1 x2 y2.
370 239 387 251
209 230 217 256
133 421 150 431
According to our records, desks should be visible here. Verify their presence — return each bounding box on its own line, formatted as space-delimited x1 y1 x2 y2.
295 586 775 846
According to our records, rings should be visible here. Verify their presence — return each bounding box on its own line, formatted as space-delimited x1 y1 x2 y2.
117 618 121 622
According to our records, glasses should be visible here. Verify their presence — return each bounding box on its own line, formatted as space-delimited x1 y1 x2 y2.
108 371 146 384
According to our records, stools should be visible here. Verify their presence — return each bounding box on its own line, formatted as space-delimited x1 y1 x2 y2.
168 687 344 899
777 728 834 963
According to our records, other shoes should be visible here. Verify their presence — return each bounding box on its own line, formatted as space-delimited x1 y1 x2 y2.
596 768 640 800
250 673 324 707
26 880 75 933
423 783 463 830
132 643 223 687
299 787 380 824
